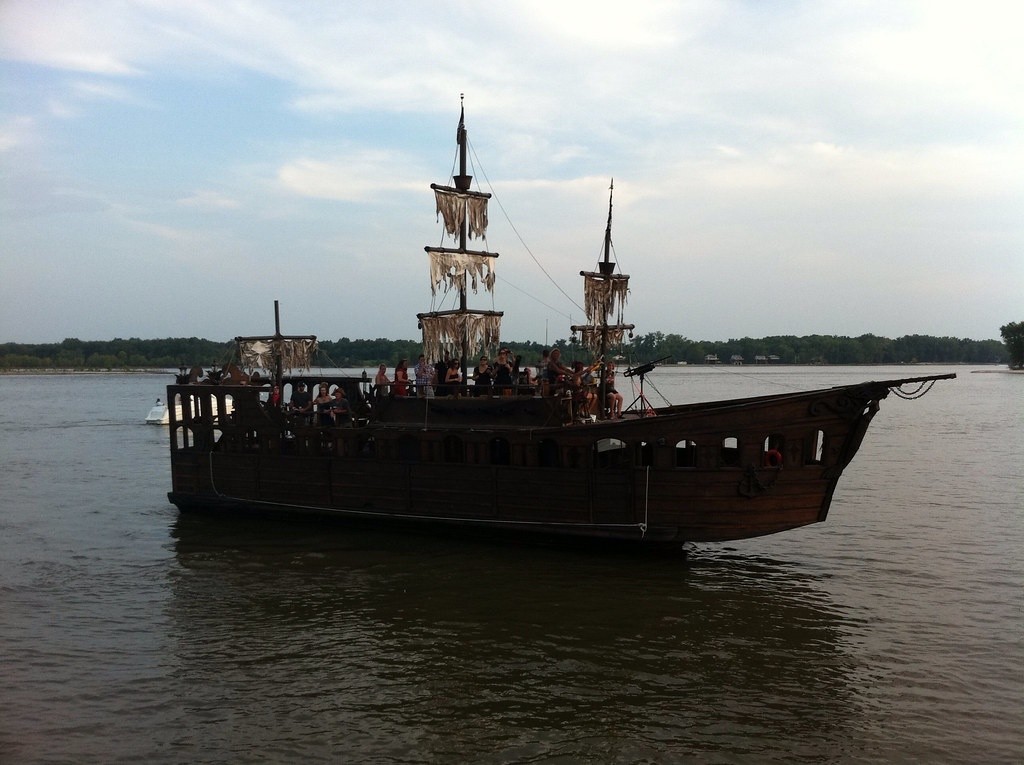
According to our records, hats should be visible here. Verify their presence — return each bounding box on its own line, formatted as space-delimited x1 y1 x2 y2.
331 387 346 396
298 381 306 387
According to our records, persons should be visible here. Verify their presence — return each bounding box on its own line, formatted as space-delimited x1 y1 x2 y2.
312 382 352 427
472 347 516 397
375 364 390 397
434 350 452 396
525 348 624 426
289 382 313 414
394 359 412 396
414 354 438 397
445 358 462 396
266 385 281 408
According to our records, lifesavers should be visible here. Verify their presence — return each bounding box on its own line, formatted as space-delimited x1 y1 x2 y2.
765 449 782 469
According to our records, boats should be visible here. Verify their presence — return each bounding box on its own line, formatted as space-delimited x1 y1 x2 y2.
163 93 958 550
145 394 233 425
